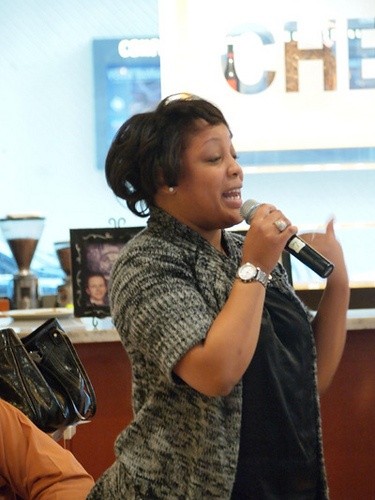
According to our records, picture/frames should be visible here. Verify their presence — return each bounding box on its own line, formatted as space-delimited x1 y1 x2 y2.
69 226 146 318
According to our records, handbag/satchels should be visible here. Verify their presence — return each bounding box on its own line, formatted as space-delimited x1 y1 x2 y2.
0 318 97 433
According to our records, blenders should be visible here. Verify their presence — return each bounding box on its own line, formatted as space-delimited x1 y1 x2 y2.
54 242 72 307
0 215 46 307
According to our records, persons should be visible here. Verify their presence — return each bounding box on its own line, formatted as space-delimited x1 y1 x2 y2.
0 399 97 500
86 93 350 500
84 273 108 307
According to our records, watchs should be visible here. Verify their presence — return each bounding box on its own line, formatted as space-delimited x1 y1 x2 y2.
236 262 273 287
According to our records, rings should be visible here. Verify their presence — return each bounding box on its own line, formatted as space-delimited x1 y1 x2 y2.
274 218 288 232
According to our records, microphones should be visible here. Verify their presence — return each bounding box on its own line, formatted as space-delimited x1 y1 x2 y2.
239 199 334 279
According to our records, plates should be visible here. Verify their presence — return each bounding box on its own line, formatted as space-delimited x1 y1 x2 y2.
0 308 74 318
